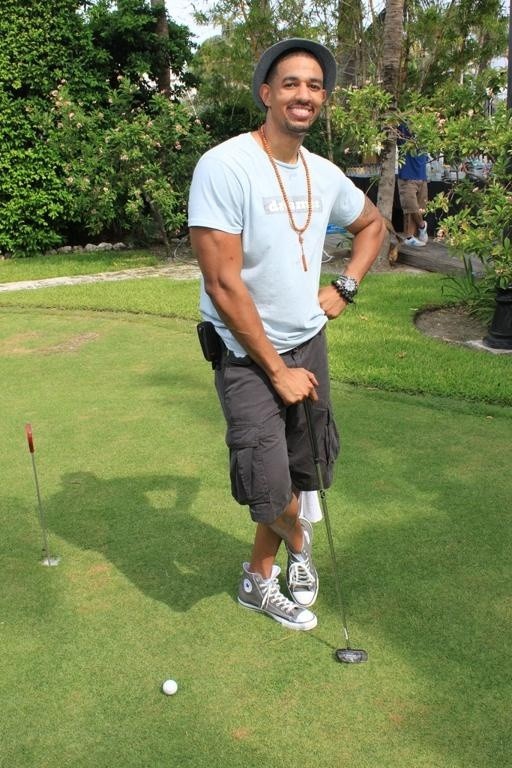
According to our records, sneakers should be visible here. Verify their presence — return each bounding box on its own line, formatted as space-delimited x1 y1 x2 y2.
284 517 319 606
238 562 317 630
405 221 429 246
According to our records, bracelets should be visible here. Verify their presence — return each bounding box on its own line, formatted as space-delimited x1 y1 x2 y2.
331 280 353 303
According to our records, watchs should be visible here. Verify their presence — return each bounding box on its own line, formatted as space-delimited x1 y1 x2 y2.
337 275 358 297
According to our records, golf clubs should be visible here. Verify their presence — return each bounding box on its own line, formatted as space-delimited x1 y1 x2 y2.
304 397 368 663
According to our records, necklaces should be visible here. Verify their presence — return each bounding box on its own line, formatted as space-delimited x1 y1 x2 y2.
258 123 312 271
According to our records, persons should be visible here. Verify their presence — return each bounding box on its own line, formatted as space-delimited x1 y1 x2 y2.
188 37 388 631
397 125 428 247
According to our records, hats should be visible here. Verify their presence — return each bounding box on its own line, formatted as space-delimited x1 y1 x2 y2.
252 38 336 112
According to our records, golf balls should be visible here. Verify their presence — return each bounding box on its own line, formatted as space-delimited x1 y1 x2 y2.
162 680 178 695
